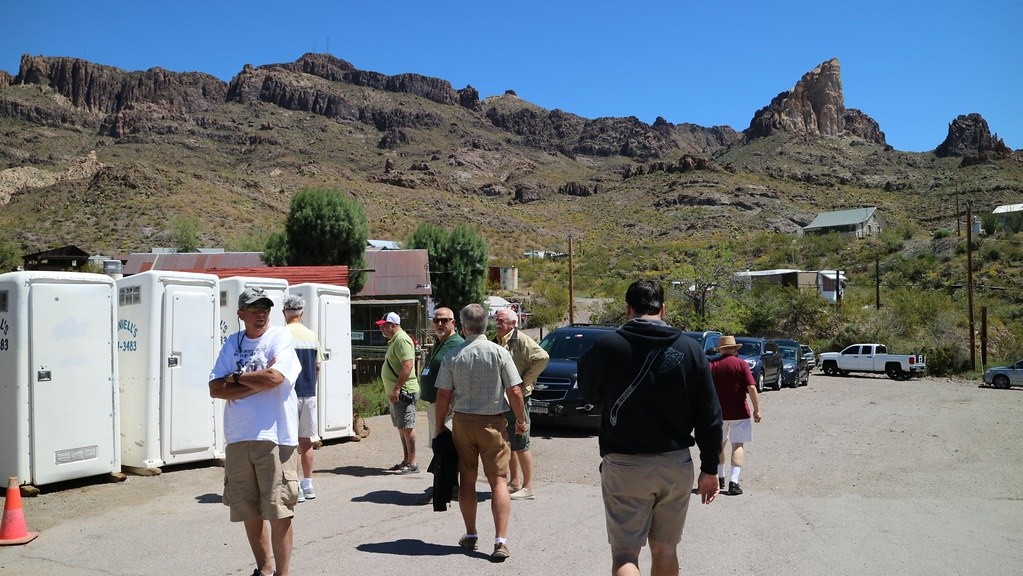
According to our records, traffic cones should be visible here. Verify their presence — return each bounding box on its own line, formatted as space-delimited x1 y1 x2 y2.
0 477 39 545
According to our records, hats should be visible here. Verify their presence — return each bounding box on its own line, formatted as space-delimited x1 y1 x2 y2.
375 312 400 325
238 287 274 309
713 336 743 351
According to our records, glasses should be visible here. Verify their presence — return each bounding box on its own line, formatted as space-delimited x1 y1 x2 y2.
433 318 453 324
247 304 271 313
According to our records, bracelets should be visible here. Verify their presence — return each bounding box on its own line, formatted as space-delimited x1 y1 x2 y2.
516 419 526 427
233 370 243 383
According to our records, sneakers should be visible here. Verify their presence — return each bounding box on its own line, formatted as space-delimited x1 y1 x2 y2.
297 481 316 502
458 534 479 550
491 542 510 558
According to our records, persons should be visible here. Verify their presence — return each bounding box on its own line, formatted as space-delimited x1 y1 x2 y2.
375 311 420 474
491 309 550 499
577 278 723 576
209 286 302 576
414 307 465 504
709 335 761 494
434 304 529 557
282 294 325 501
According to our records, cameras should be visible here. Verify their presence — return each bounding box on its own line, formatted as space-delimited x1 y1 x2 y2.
398 389 413 404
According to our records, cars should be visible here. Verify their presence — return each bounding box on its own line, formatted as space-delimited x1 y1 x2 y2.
773 339 809 388
800 344 815 371
984 359 1023 389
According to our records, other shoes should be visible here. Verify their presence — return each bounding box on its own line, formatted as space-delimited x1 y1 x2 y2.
728 481 743 495
719 477 726 489
507 484 535 500
389 461 420 475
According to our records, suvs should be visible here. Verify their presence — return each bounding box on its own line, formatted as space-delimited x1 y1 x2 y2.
734 336 783 393
528 322 620 435
683 330 724 362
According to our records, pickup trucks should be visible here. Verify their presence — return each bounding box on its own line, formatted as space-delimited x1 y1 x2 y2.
819 344 925 381
